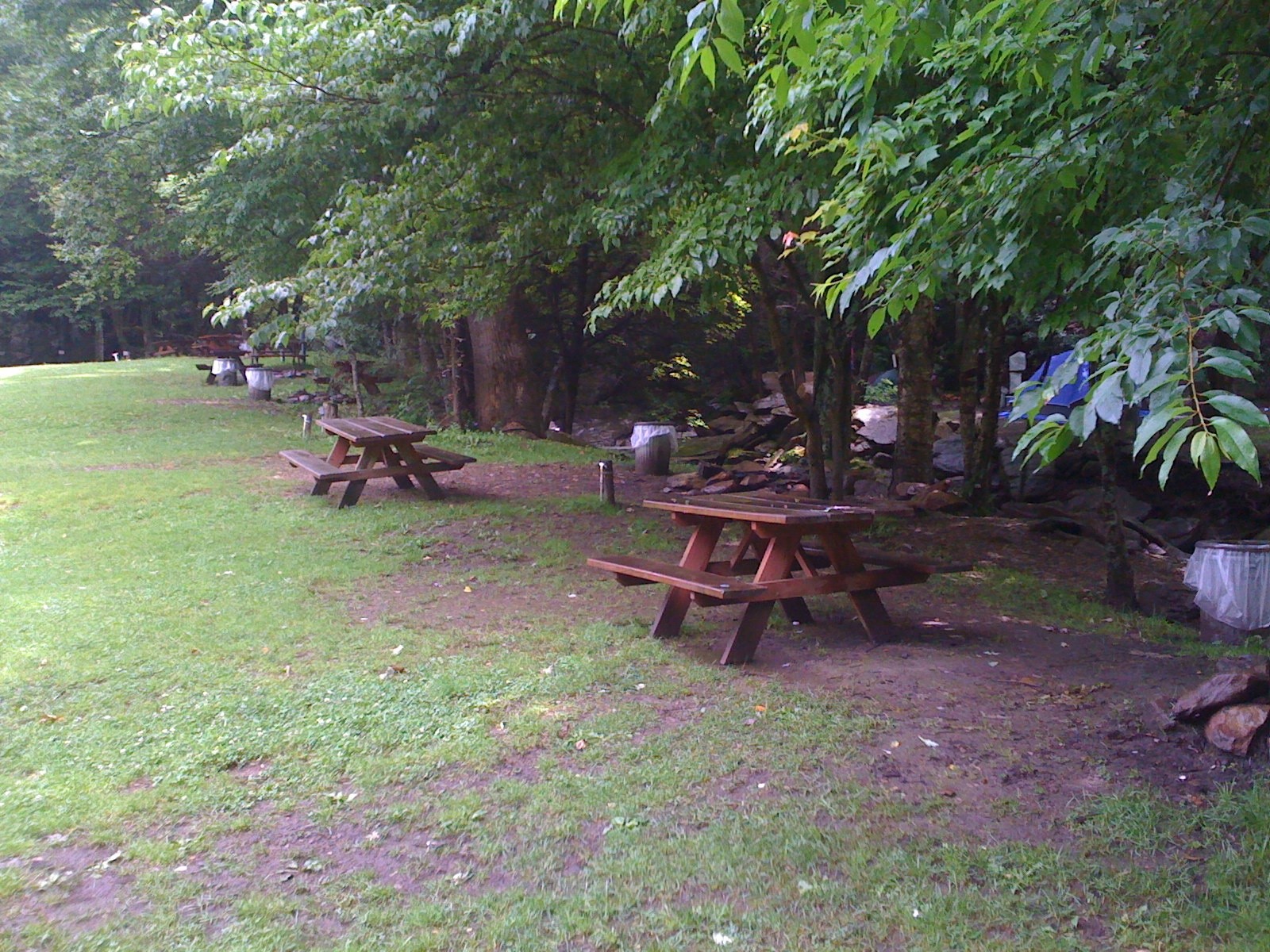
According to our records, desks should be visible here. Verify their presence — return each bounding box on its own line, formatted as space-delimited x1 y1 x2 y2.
198 333 235 356
206 348 247 385
326 360 381 398
644 493 915 666
363 372 393 383
150 339 179 356
309 415 446 510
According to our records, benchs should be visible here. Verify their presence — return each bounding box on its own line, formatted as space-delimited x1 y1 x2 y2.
412 444 477 465
196 365 212 370
244 363 264 369
800 540 973 574
151 350 176 356
260 350 286 356
191 345 210 350
278 449 340 479
586 554 767 599
252 354 308 366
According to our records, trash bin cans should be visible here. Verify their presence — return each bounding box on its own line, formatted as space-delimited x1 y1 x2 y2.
215 358 237 386
634 422 676 474
246 367 273 401
1196 541 1270 644
